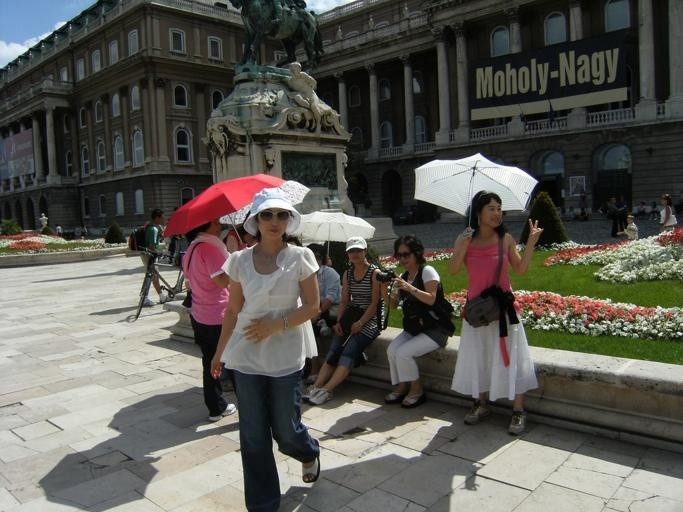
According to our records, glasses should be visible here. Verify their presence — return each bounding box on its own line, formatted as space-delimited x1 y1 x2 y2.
258 208 290 225
394 251 415 260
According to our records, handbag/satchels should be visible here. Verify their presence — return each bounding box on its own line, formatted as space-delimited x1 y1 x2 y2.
463 286 506 328
401 295 454 335
340 303 365 335
182 287 194 307
665 214 678 226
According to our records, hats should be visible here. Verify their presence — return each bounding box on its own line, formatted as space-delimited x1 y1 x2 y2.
243 188 303 238
345 237 368 252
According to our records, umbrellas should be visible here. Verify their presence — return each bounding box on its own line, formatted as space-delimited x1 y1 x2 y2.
289 210 376 257
413 152 539 227
219 180 311 226
162 174 288 245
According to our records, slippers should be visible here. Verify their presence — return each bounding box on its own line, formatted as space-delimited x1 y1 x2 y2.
301 438 321 484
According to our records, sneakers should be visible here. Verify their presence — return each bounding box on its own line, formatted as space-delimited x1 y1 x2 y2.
208 403 236 422
299 385 322 401
138 298 157 306
464 401 529 436
309 389 335 406
158 294 172 303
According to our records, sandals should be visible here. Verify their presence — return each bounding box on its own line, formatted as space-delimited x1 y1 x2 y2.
400 388 427 409
383 387 410 404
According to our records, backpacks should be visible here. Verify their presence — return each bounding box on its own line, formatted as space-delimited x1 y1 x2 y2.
126 220 151 252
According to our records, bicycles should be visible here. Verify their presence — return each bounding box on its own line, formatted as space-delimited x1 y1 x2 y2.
130 244 189 320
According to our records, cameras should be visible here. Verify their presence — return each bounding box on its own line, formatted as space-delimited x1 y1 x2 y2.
377 267 395 285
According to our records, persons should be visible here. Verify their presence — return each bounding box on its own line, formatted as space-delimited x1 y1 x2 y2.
448 190 544 435
80 222 88 240
181 217 245 422
140 207 188 306
210 187 320 512
55 223 63 237
281 236 455 408
223 226 256 255
559 193 678 241
